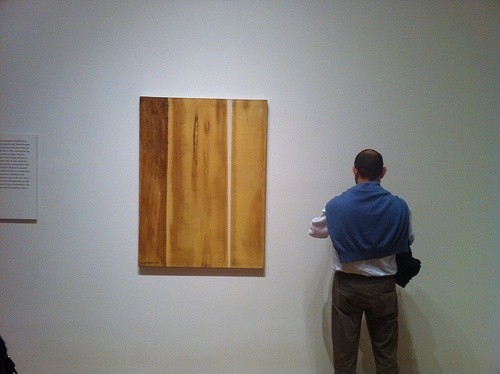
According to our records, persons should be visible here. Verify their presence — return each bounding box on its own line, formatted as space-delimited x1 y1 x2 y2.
308 149 421 374
